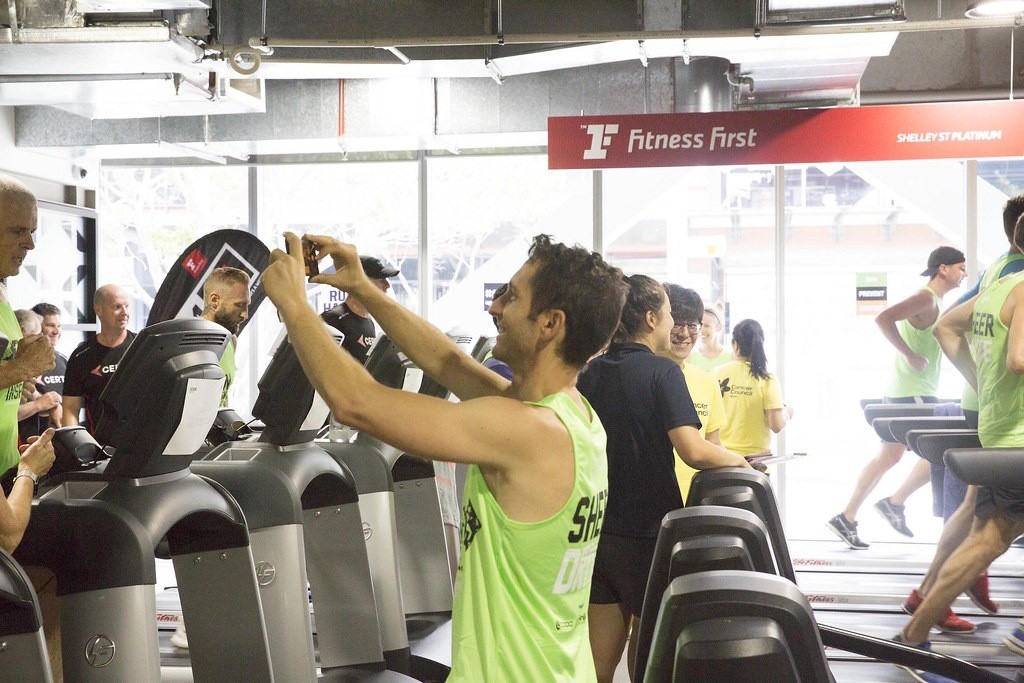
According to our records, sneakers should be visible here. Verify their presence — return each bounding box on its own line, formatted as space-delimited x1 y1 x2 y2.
964 569 997 614
874 497 913 537
903 589 977 634
826 513 869 549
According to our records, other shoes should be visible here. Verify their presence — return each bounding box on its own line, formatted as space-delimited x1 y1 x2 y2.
1003 619 1024 656
171 620 189 649
891 634 959 683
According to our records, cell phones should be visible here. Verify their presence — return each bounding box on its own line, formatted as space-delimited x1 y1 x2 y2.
285 238 319 276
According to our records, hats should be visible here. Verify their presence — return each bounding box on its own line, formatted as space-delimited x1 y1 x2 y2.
359 256 400 278
920 246 965 276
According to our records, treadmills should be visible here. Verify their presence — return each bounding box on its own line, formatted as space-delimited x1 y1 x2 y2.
634 398 1024 683
0 319 497 683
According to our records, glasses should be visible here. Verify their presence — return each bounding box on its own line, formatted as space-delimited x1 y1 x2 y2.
671 319 703 335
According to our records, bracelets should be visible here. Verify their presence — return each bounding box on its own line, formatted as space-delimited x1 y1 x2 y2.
13 470 39 485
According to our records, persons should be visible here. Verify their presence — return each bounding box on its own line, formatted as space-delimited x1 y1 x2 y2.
62 285 138 427
661 284 793 509
827 247 968 550
196 266 250 409
0 428 56 555
894 212 1024 652
904 198 1024 631
0 174 70 500
261 232 628 682
571 274 754 682
319 256 400 364
482 284 511 381
